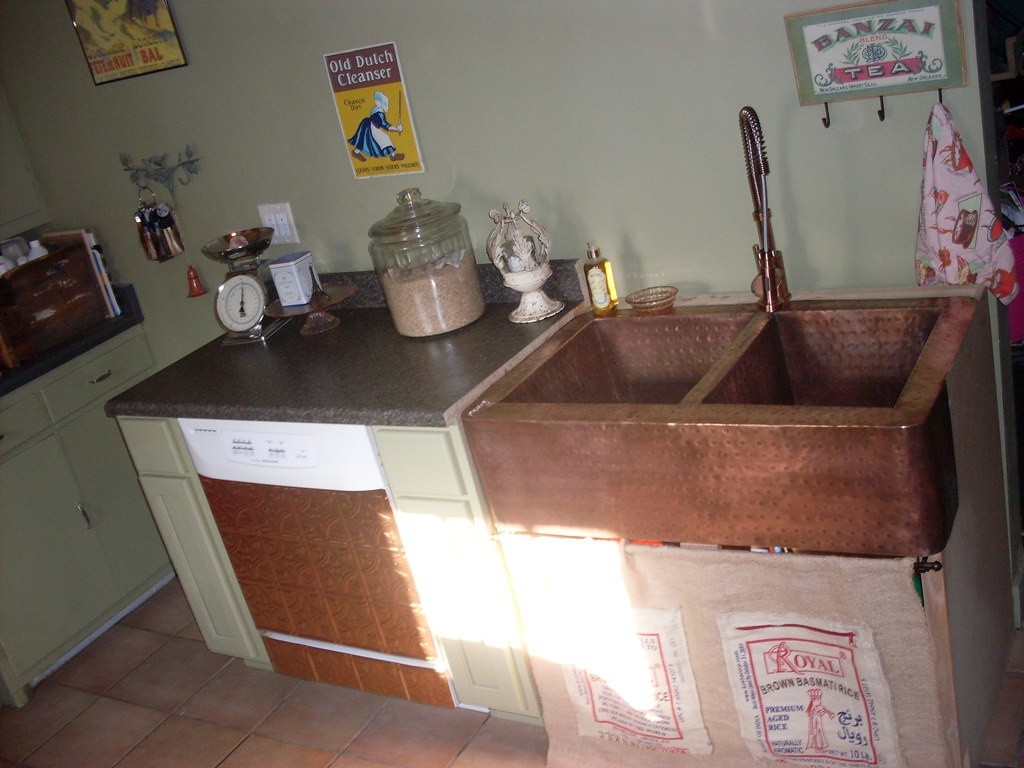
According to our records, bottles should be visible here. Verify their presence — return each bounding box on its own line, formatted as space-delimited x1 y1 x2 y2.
369 190 485 339
0 240 49 277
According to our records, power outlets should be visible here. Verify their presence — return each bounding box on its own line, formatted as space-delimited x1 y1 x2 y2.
258 203 299 245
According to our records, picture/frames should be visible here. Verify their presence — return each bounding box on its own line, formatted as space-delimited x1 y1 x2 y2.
66 0 188 85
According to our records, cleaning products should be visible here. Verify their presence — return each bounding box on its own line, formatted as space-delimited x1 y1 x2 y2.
584 240 619 316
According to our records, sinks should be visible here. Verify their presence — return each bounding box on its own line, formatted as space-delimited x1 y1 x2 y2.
460 305 756 425
701 294 980 427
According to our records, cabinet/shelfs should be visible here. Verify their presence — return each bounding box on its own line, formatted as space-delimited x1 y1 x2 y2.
114 415 273 671
0 323 176 710
372 424 544 727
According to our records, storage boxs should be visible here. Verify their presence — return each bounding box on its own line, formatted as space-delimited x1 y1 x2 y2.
268 251 323 307
0 239 110 372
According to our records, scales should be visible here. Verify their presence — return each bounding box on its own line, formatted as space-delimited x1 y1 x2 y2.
200 225 293 349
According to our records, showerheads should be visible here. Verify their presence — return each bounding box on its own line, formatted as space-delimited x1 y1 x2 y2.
755 247 783 314
